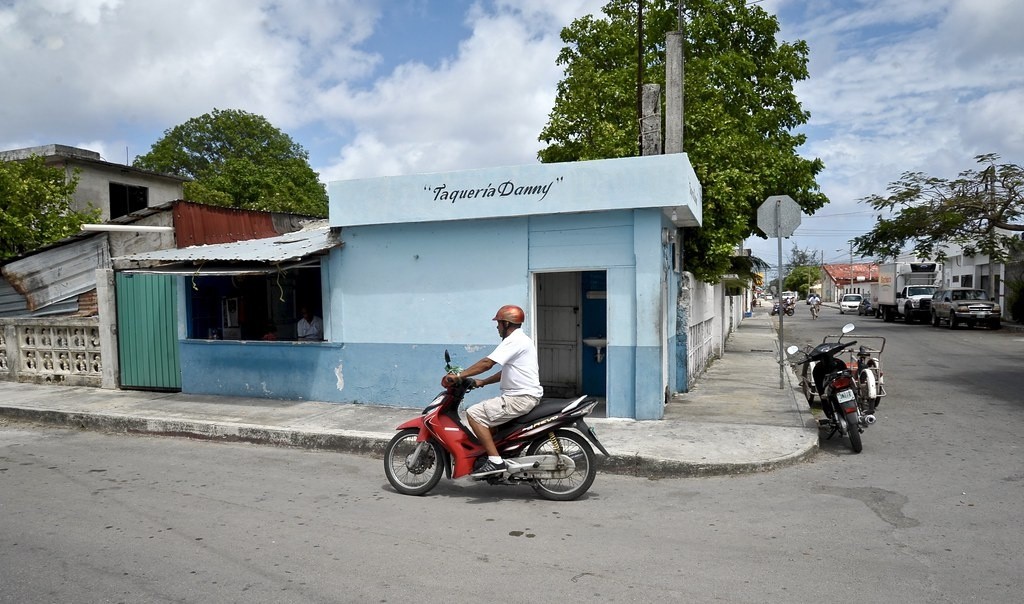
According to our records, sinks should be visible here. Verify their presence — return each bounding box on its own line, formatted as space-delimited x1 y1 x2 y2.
582 336 606 348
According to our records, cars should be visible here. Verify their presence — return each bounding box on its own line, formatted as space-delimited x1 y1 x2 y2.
858 297 876 317
760 292 773 300
806 293 822 305
838 293 864 315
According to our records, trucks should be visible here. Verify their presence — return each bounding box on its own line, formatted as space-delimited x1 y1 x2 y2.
782 291 795 302
870 283 880 319
878 262 943 324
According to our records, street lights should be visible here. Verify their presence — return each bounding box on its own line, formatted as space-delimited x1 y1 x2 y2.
836 248 853 294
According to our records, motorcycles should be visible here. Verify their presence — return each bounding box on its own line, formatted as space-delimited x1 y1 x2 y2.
786 322 877 454
770 301 794 316
384 349 610 500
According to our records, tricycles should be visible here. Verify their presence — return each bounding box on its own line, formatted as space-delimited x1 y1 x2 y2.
798 334 887 415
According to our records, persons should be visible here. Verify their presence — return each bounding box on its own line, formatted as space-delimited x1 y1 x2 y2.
445 304 542 474
808 294 821 311
296 305 323 340
262 322 279 340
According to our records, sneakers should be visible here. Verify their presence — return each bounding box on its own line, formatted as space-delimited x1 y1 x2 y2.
470 459 508 477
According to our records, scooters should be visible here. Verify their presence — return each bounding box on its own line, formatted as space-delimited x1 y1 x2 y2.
807 301 822 320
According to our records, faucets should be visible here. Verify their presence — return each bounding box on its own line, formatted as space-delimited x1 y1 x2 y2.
597 332 603 339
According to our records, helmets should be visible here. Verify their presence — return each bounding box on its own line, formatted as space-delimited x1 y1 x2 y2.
492 305 524 324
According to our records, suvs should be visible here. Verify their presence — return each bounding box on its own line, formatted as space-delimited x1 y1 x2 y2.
928 287 1002 331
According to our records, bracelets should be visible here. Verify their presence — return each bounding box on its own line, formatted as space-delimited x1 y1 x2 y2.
456 371 463 381
